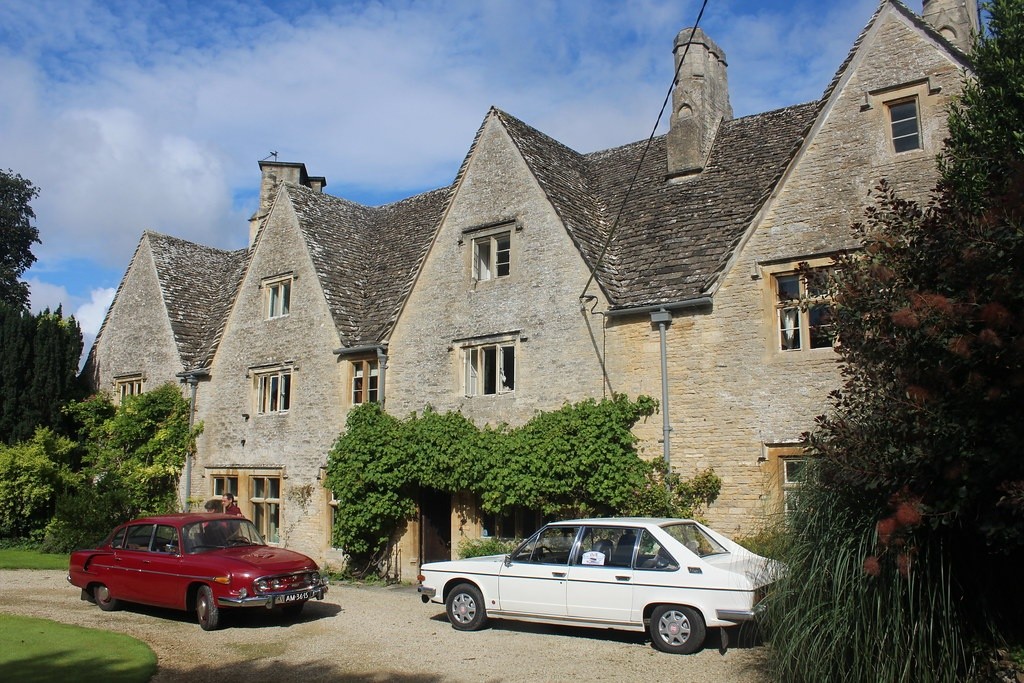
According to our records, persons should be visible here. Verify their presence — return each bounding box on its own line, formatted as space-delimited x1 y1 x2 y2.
201 499 228 539
221 492 245 541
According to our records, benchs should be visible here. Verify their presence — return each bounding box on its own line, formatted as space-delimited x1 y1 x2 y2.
115 537 171 555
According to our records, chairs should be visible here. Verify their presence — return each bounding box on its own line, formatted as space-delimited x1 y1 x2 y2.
193 533 218 554
578 540 616 567
609 533 636 564
637 546 665 568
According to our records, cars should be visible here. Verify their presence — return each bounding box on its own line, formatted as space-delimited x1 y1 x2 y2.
416 515 788 656
65 511 329 631
